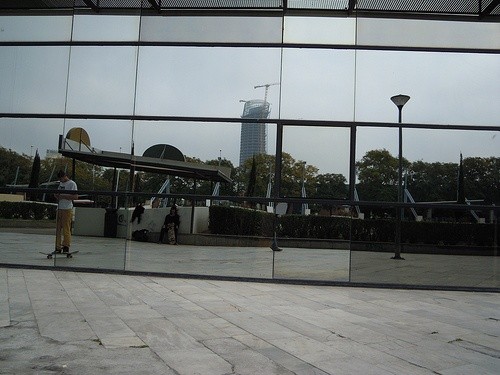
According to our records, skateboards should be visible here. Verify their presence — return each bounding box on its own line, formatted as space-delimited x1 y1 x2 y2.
39 250 79 259
167 223 176 244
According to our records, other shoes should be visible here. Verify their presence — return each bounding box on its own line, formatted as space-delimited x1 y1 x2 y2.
62 246 69 254
53 249 61 253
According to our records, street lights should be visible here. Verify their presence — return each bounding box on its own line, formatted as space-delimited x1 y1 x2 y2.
391 95 410 260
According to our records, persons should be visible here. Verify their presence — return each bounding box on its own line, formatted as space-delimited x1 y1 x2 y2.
158 205 180 245
53 170 78 253
